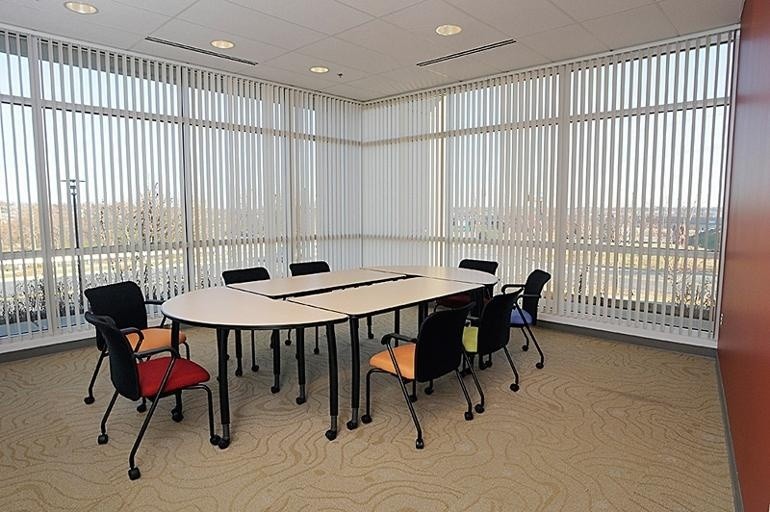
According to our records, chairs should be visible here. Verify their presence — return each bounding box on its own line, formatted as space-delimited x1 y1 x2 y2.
85 311 221 481
433 259 497 325
285 261 374 354
361 301 477 450
222 266 299 371
462 284 524 413
501 269 552 369
84 280 189 412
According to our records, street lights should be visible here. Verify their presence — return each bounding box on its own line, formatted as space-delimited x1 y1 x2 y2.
60 177 87 314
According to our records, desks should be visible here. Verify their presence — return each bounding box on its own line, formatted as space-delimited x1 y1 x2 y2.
161 262 497 449
